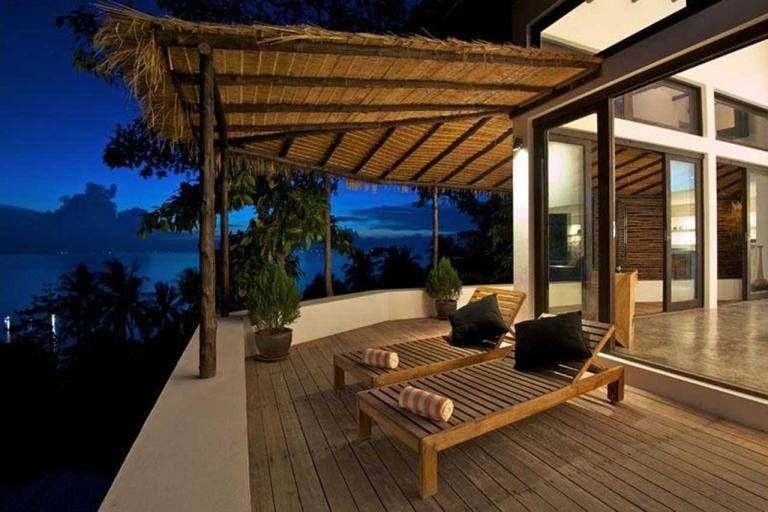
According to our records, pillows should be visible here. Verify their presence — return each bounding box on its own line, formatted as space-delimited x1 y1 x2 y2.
396 383 455 423
445 292 510 347
511 308 594 371
360 346 401 371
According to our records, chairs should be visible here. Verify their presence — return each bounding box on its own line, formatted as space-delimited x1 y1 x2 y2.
353 311 628 498
332 290 528 410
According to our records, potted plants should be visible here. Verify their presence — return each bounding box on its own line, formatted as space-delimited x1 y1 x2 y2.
234 261 302 363
423 254 468 322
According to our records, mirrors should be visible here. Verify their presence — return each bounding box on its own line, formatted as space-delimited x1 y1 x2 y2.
740 164 766 302
547 132 593 283
662 153 704 314
